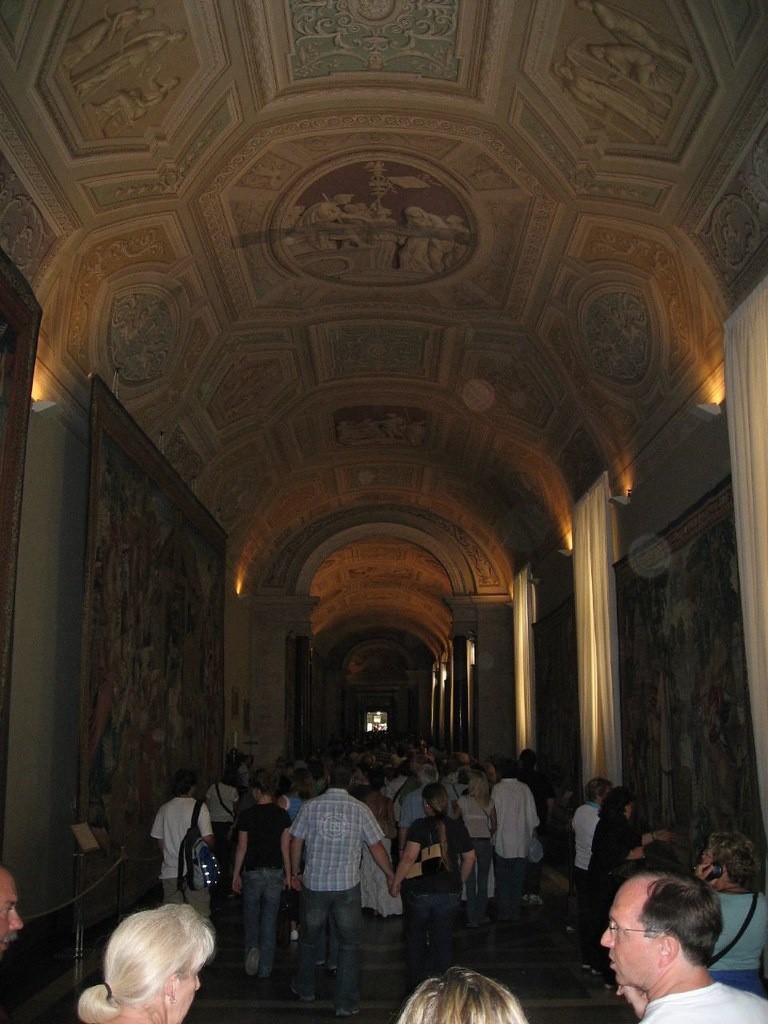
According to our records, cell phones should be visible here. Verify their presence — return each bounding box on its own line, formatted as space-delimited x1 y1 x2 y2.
702 863 723 881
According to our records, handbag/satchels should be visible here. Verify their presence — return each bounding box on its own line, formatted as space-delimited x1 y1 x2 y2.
402 818 442 881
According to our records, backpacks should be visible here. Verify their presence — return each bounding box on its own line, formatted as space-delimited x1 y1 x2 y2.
178 801 220 897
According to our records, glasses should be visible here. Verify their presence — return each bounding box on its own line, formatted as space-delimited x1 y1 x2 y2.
609 922 660 934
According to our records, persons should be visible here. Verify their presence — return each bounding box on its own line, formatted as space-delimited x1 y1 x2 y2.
0 865 24 960
75 903 218 1024
393 962 528 1024
146 722 673 996
616 979 650 1019
686 830 767 1000
601 868 768 1024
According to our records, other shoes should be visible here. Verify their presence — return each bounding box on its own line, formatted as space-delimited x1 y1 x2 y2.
247 946 270 979
465 915 489 927
337 1004 359 1018
496 912 517 923
291 985 315 1001
521 893 545 906
